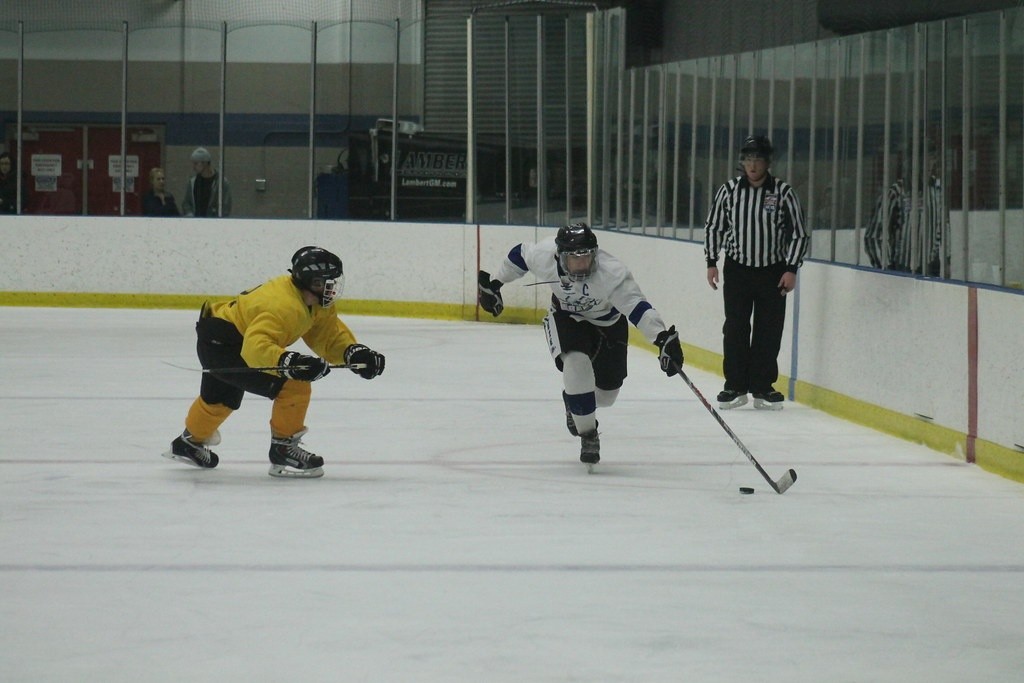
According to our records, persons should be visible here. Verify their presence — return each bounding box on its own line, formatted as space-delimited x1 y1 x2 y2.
142 167 180 217
479 223 684 474
164 245 386 477
864 136 952 278
704 135 811 409
181 148 232 218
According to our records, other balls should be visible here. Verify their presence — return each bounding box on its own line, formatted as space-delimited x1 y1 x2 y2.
739 487 755 495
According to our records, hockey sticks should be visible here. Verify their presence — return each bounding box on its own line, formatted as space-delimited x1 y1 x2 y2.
673 360 799 496
159 357 368 375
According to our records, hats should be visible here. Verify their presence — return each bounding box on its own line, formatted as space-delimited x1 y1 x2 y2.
190 147 210 162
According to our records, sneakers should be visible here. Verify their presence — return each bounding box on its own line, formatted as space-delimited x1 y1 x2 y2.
563 389 581 435
717 386 749 411
753 385 785 411
268 421 324 478
161 426 220 468
580 418 600 475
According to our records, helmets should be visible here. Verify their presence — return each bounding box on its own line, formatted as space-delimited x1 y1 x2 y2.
555 221 599 282
291 245 345 309
742 135 772 161
910 136 936 154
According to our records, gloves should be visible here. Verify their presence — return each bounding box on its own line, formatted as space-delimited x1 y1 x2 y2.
478 270 504 316
344 342 385 379
278 352 331 382
653 325 684 377
